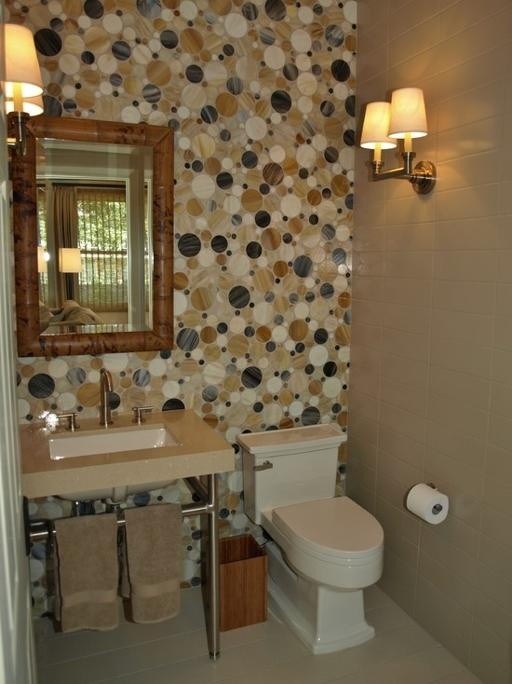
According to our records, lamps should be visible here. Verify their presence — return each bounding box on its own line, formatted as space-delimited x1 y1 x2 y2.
360 88 436 196
1 21 45 156
52 244 88 301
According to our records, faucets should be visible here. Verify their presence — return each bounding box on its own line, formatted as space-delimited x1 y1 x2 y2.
99 365 116 427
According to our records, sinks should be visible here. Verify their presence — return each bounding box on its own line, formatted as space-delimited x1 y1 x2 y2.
44 421 185 462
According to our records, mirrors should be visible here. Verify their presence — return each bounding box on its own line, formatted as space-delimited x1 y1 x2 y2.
12 116 173 356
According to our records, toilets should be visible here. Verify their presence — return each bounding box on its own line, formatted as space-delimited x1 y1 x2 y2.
235 422 385 656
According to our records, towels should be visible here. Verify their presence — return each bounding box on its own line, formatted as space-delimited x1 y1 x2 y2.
124 504 183 624
52 515 120 632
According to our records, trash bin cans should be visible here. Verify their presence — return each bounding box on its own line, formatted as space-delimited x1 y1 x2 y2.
200 533 268 632
